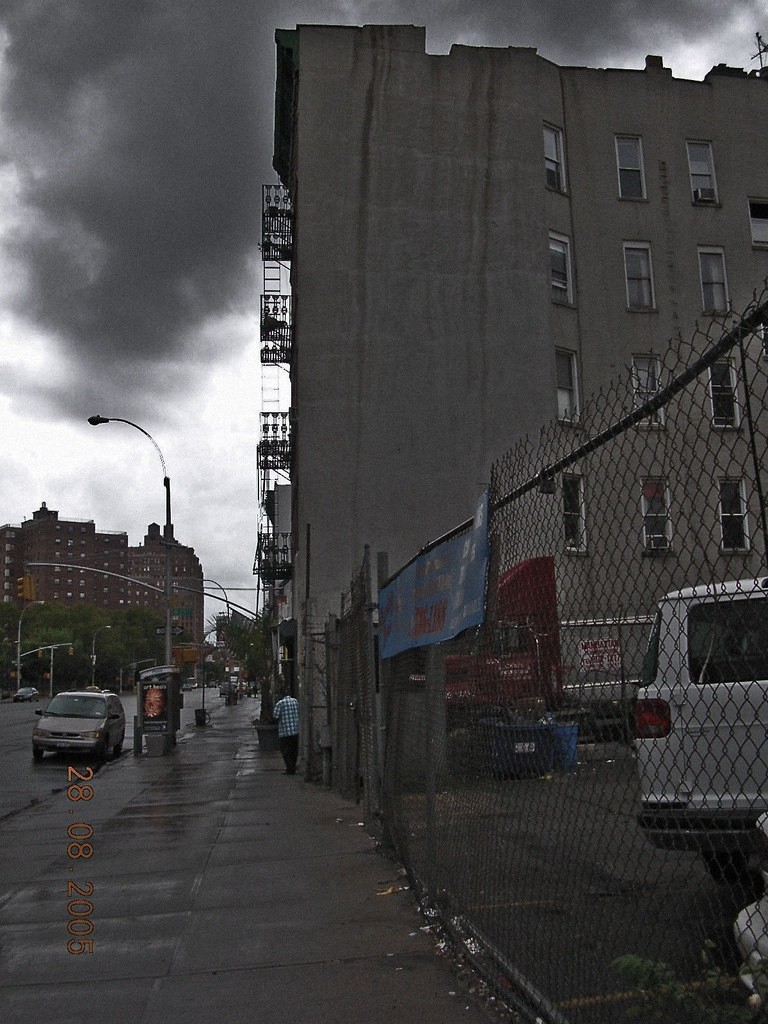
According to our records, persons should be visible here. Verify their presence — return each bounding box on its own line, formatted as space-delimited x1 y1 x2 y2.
253 685 257 698
273 689 299 774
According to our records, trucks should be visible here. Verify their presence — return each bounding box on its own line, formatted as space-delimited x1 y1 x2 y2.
447 616 652 739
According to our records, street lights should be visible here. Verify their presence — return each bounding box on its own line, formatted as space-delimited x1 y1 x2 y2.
90 625 111 686
16 601 45 691
87 415 175 751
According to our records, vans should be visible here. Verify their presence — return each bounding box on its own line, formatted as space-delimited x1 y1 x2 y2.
632 578 768 882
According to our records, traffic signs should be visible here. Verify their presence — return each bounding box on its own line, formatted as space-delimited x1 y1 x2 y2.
157 627 183 636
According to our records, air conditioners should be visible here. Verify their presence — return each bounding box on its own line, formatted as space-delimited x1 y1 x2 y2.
694 188 716 202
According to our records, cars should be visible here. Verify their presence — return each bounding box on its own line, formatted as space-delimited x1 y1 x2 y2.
31 686 127 762
13 686 39 703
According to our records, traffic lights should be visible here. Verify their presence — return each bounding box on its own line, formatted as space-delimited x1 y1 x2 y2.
69 646 74 657
17 575 35 601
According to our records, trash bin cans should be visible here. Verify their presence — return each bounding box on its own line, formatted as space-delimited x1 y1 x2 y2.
471 718 580 784
